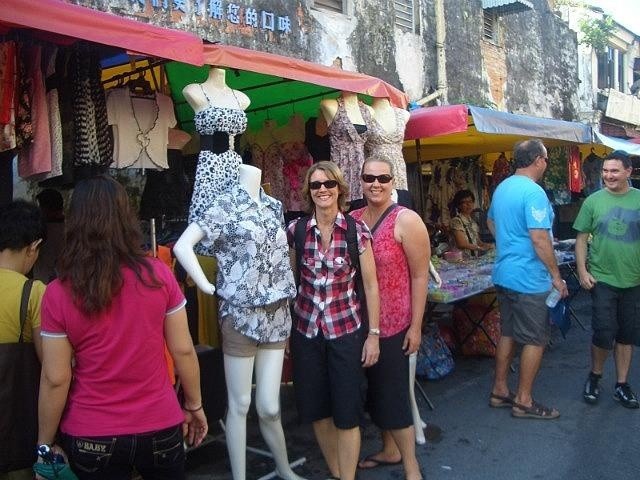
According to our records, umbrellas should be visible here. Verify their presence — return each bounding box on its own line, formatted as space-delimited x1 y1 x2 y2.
33 444 76 480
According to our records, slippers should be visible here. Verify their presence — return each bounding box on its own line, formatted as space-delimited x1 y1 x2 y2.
359 454 402 470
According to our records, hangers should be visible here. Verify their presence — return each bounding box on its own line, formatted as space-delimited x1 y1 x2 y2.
586 148 600 162
113 66 152 93
602 151 608 158
3 29 57 50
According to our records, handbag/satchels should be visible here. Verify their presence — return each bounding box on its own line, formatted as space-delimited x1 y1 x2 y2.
416 320 456 381
457 296 502 357
0 341 42 472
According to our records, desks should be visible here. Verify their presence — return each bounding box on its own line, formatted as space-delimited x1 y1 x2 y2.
425 241 587 373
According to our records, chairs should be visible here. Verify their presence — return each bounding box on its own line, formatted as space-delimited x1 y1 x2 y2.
175 348 307 480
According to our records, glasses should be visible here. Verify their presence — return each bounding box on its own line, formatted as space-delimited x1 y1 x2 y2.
362 174 392 183
309 180 337 188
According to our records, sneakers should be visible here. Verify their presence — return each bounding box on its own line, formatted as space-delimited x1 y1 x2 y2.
583 371 602 400
612 382 640 408
489 389 560 419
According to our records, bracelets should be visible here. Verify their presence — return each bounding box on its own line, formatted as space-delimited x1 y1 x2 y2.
476 246 480 251
181 402 205 414
368 329 382 336
44 441 55 448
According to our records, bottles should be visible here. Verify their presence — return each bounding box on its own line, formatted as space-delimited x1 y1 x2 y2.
544 280 566 309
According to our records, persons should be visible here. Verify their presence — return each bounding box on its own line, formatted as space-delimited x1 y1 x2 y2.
36 175 209 479
347 154 431 480
485 138 569 419
32 189 68 282
450 189 493 258
284 161 381 480
173 164 300 480
0 199 48 477
242 89 413 217
573 151 640 408
183 67 252 256
